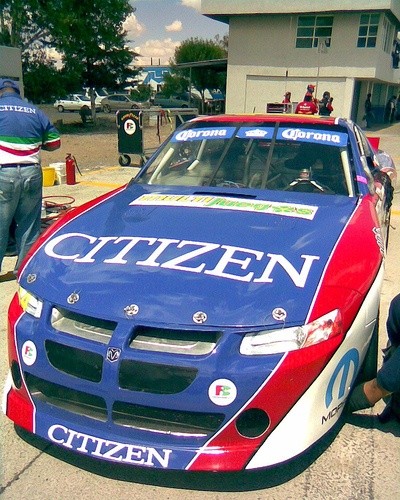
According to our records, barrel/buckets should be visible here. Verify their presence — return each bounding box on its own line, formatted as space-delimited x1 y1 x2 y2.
43 167 56 187
367 137 379 150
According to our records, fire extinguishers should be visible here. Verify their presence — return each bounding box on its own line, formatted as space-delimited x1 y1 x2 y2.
65 153 82 185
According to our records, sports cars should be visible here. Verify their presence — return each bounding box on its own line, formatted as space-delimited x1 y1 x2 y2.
2 115 397 474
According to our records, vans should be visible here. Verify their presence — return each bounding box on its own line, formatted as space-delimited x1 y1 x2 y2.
154 92 198 108
81 87 109 103
101 94 150 114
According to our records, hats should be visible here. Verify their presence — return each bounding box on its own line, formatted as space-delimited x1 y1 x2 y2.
306 92 312 96
307 85 315 88
0 79 19 94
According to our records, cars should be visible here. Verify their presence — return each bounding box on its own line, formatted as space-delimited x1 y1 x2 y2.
54 94 100 113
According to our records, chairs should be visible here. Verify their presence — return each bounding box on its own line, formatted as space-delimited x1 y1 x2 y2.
208 138 257 188
274 142 327 195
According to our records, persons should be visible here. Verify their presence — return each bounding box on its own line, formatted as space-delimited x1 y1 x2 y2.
0 79 60 278
386 96 397 124
344 294 400 423
282 92 291 111
392 39 400 69
295 85 334 115
363 93 375 128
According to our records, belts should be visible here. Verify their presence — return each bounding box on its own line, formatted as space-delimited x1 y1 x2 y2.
0 163 40 168
